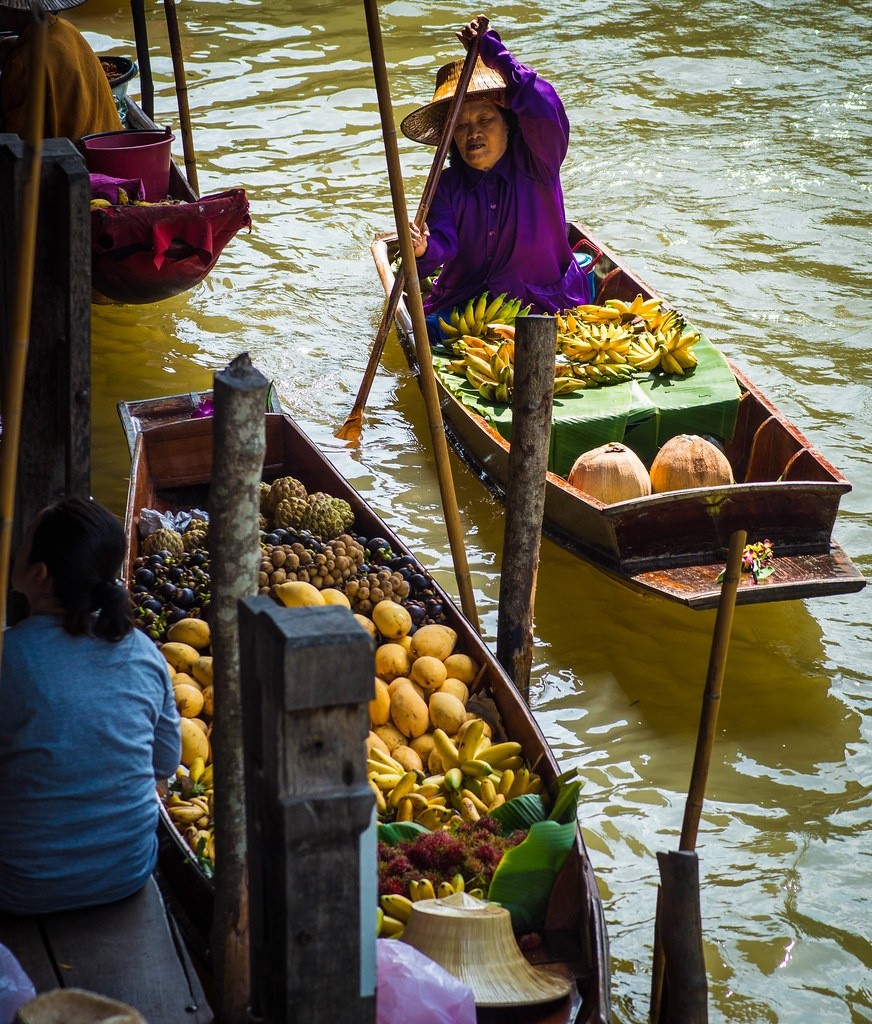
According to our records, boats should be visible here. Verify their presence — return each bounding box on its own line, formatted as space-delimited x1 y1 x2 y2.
112 384 616 1023
368 217 871 611
75 78 254 307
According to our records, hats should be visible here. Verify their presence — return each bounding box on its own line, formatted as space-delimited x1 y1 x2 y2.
400 56 509 146
396 892 572 1008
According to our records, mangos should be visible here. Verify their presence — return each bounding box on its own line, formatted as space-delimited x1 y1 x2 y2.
155 582 494 771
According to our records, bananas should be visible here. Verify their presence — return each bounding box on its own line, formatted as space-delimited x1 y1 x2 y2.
397 255 441 304
438 290 702 405
167 717 544 940
90 187 152 208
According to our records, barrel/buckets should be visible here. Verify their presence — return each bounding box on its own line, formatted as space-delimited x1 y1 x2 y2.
571 239 603 305
78 123 176 204
97 54 138 105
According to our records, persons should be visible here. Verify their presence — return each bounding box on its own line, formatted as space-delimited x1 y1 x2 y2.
1 498 182 923
400 21 593 346
1 0 121 151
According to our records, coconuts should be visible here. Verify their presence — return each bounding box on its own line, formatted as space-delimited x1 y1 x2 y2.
649 434 733 493
567 442 651 505
